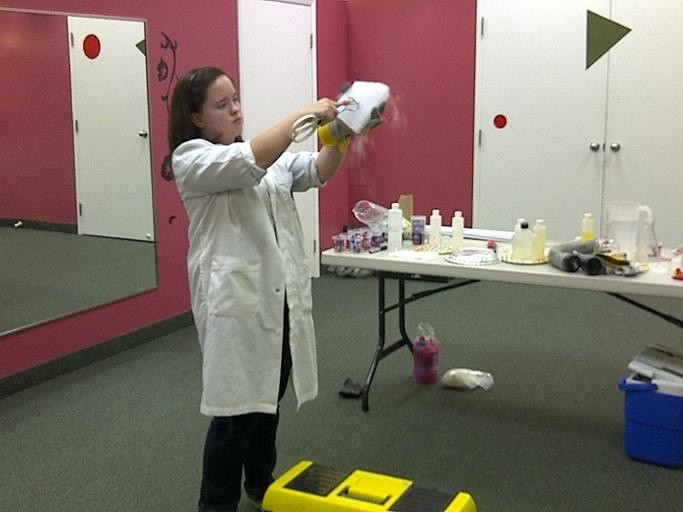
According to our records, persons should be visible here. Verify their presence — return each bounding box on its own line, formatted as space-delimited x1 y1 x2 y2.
158 67 387 511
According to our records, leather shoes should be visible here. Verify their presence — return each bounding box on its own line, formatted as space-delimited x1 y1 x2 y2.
318 81 386 153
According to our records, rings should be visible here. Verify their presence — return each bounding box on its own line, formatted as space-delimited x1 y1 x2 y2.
368 245 387 253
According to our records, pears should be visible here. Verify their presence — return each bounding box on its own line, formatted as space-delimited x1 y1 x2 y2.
509 211 547 265
386 202 465 255
580 213 595 241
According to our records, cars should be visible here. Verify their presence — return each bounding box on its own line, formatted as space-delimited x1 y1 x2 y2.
605 203 656 263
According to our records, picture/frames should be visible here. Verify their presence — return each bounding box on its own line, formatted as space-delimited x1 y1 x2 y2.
320 233 682 412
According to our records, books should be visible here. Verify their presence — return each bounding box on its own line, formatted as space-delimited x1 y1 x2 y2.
618 341 683 471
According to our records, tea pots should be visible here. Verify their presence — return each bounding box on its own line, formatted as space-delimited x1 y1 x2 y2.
1 7 160 337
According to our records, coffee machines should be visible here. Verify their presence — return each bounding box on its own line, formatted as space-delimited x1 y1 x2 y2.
290 99 342 142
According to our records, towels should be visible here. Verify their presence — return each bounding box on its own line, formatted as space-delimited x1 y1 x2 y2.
618 344 681 471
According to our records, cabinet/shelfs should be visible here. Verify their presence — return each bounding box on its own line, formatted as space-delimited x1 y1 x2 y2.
581 213 593 240
510 217 546 262
429 209 441 245
451 211 464 250
386 203 403 253
